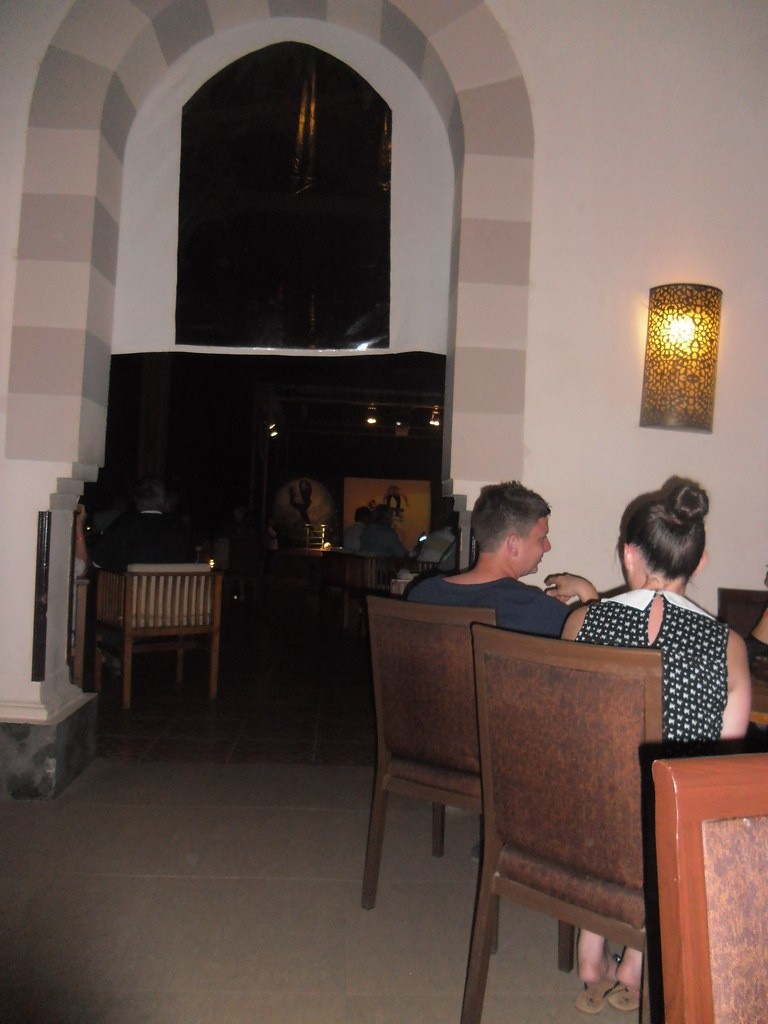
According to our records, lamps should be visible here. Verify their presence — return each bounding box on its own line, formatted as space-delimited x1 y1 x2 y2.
638 281 725 436
429 409 440 427
266 411 278 438
364 402 377 424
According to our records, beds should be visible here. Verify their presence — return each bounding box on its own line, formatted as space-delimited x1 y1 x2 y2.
90 547 225 710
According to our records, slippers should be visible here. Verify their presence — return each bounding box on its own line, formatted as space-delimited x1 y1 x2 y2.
574 954 627 1016
607 980 640 1012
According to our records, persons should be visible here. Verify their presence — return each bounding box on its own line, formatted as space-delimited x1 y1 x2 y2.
76 476 198 673
406 481 598 640
346 504 409 559
416 513 458 573
562 477 752 994
749 572 768 656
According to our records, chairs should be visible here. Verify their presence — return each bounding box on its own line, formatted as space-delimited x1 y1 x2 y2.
360 594 508 955
458 622 664 1024
652 752 767 1022
